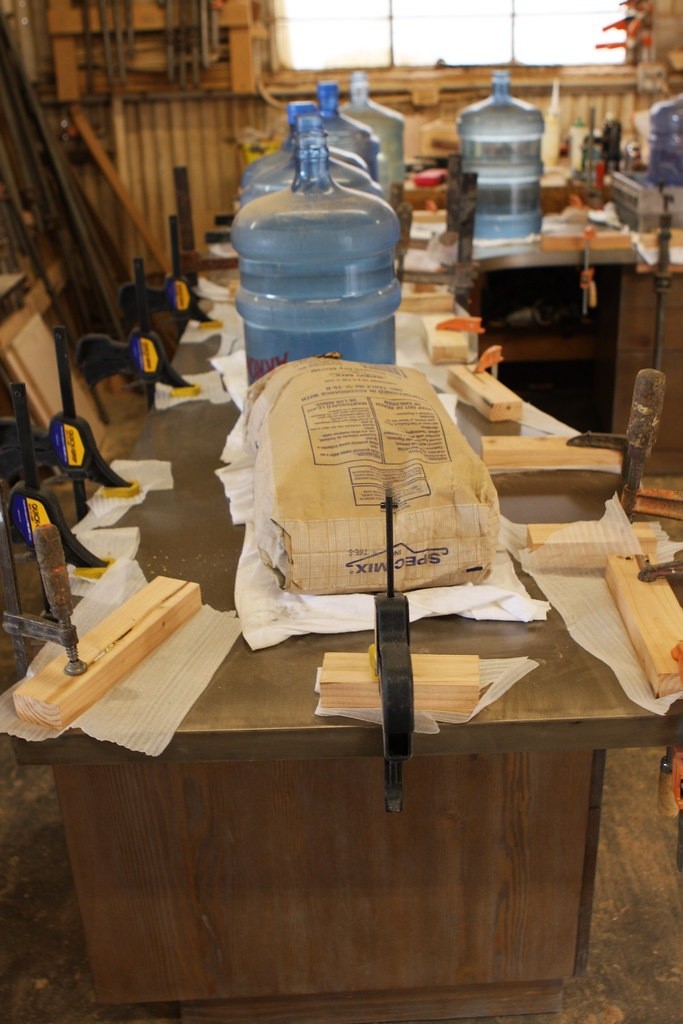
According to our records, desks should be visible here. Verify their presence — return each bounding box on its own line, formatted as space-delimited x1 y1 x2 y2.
10 166 681 1023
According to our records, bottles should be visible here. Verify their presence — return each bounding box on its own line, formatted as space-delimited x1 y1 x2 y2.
648 93 683 186
340 71 404 182
310 84 379 180
568 116 589 171
239 116 384 213
230 129 403 387
242 100 371 189
455 70 546 239
